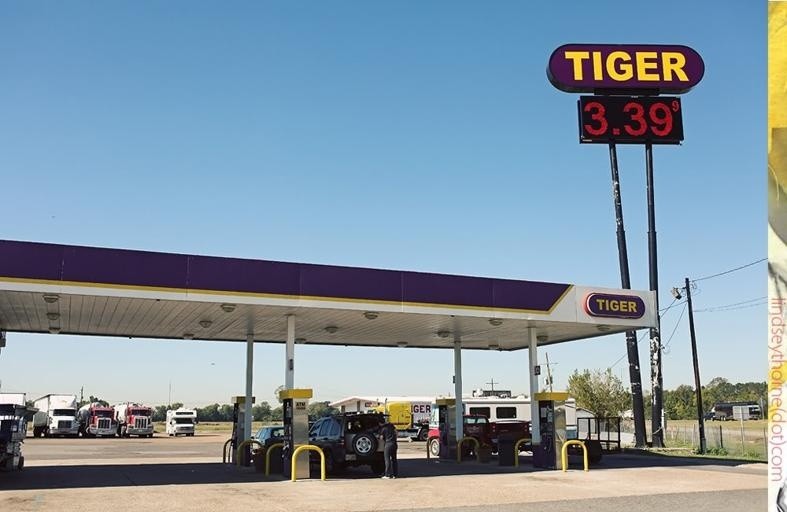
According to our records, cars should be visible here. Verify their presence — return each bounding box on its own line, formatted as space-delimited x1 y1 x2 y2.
253 426 285 442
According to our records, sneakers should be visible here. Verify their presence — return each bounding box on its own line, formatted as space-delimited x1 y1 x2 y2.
381 476 397 479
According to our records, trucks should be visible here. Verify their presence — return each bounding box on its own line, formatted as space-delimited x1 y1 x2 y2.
1 392 27 436
367 401 432 441
32 394 197 438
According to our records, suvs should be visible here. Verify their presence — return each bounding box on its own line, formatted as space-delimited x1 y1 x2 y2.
309 410 385 474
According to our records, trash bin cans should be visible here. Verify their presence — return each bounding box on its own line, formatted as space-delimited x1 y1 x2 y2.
497 432 516 465
265 437 284 473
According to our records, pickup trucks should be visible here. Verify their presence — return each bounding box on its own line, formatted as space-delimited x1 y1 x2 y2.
428 414 529 456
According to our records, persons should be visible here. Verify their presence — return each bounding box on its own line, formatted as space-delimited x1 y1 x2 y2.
377 417 398 479
